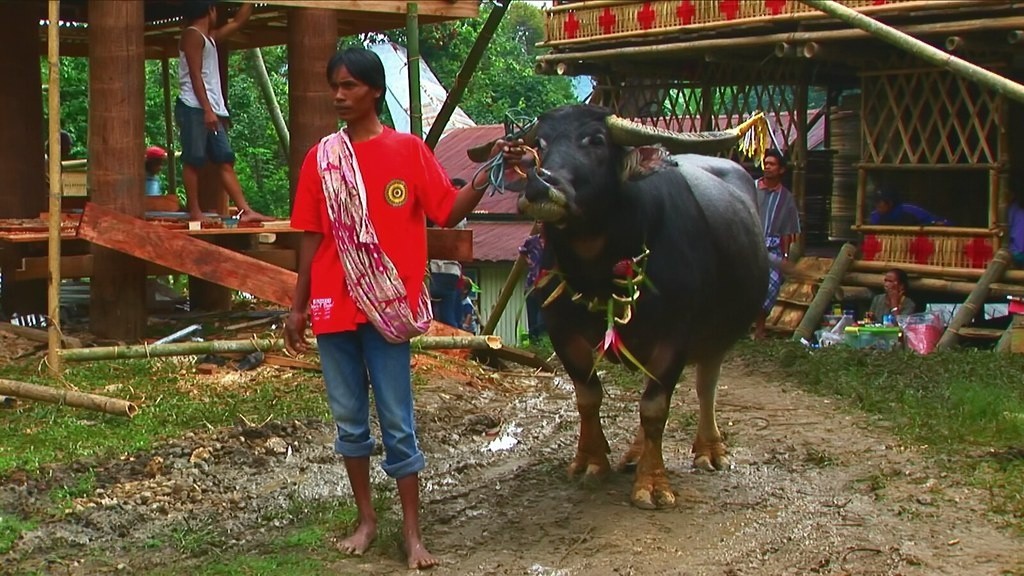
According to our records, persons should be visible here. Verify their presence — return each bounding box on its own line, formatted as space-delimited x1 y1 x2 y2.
868 187 954 228
426 178 479 333
754 149 801 340
1006 197 1024 271
869 268 917 328
175 1 276 222
282 48 524 569
145 146 165 196
45 131 72 158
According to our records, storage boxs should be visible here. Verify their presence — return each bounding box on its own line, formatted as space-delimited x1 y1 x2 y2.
844 327 900 349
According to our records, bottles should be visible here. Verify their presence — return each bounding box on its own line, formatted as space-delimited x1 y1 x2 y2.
813 303 900 349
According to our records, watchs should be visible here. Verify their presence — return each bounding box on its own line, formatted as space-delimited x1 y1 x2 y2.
783 252 789 258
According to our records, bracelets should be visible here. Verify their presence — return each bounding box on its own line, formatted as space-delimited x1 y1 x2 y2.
891 306 899 313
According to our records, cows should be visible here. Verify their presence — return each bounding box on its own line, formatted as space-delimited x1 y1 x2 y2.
465 102 769 512
